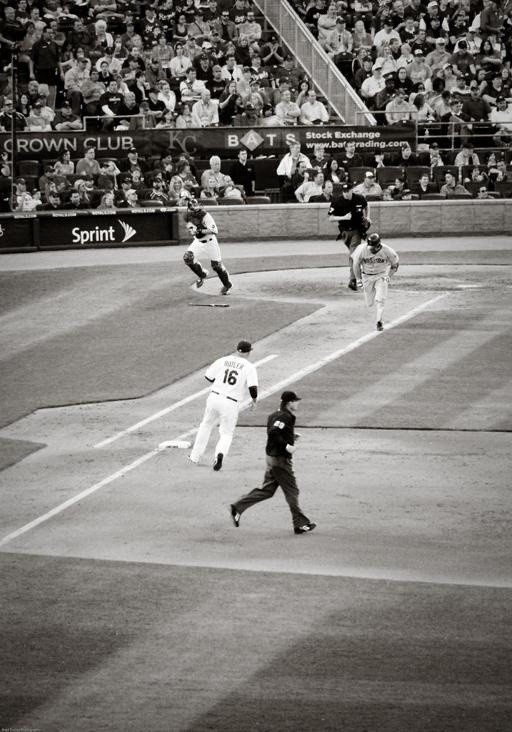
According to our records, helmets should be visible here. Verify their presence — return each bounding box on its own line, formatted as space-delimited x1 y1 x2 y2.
188 199 202 211
367 233 380 250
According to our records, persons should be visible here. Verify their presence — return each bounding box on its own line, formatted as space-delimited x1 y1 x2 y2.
328 184 372 290
183 199 232 295
1 0 511 213
190 341 258 470
230 392 316 534
350 233 399 331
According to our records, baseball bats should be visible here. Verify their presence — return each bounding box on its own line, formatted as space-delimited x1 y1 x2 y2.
188 303 229 308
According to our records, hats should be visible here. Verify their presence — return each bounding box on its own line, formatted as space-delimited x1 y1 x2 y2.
336 0 508 105
445 169 457 178
364 171 376 179
201 41 213 49
281 391 302 402
148 85 161 94
195 9 205 16
341 183 352 190
237 340 253 353
31 93 46 109
14 146 173 197
462 142 474 149
429 142 440 149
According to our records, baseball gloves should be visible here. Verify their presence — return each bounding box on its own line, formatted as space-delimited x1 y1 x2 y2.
360 217 371 233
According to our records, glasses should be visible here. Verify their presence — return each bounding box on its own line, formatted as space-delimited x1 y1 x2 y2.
4 103 12 106
480 190 489 193
222 14 230 17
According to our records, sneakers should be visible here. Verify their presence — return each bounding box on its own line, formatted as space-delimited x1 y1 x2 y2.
377 321 384 332
221 283 232 295
229 505 242 528
213 452 224 471
187 455 200 464
196 271 206 288
294 523 316 535
349 278 357 289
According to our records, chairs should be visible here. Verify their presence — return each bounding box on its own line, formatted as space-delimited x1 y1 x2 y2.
0 1 511 220
